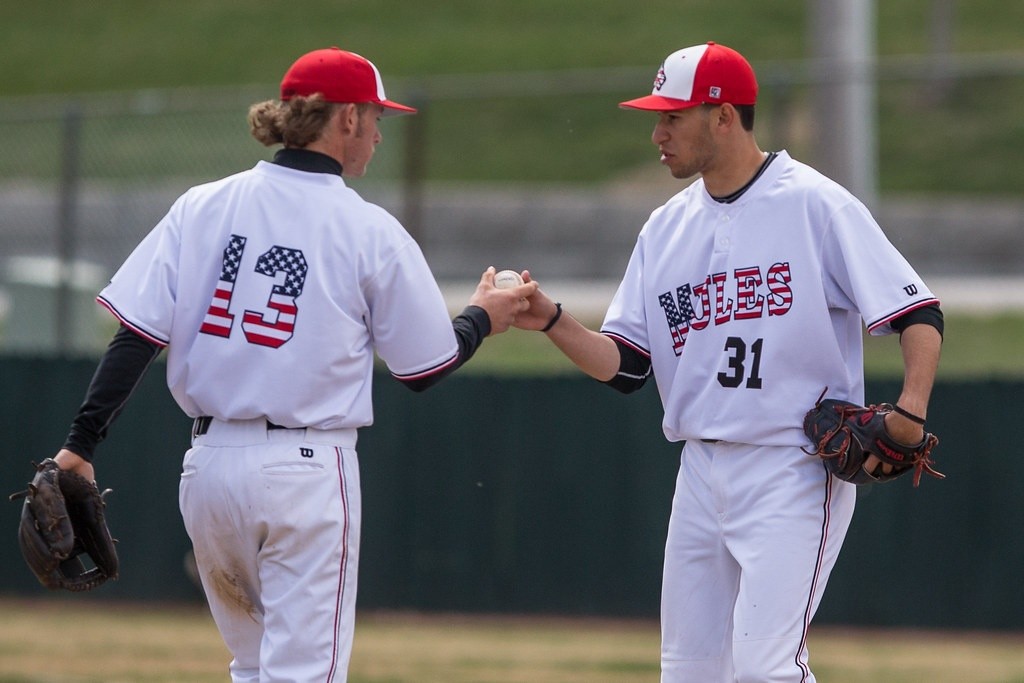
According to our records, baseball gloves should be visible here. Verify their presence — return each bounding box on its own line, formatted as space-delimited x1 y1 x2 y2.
17 458 121 592
803 398 940 486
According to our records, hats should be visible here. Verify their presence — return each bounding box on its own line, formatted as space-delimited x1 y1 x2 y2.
280 47 417 115
618 41 758 110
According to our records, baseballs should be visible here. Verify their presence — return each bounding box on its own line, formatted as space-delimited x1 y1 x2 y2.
494 269 526 289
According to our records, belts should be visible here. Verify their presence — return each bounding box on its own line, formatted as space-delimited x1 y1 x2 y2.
196 413 305 434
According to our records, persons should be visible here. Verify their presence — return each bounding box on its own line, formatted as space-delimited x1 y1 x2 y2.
490 41 943 683
11 47 539 683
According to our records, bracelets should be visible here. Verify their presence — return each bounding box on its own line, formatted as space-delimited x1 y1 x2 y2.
539 303 563 331
893 405 925 426
